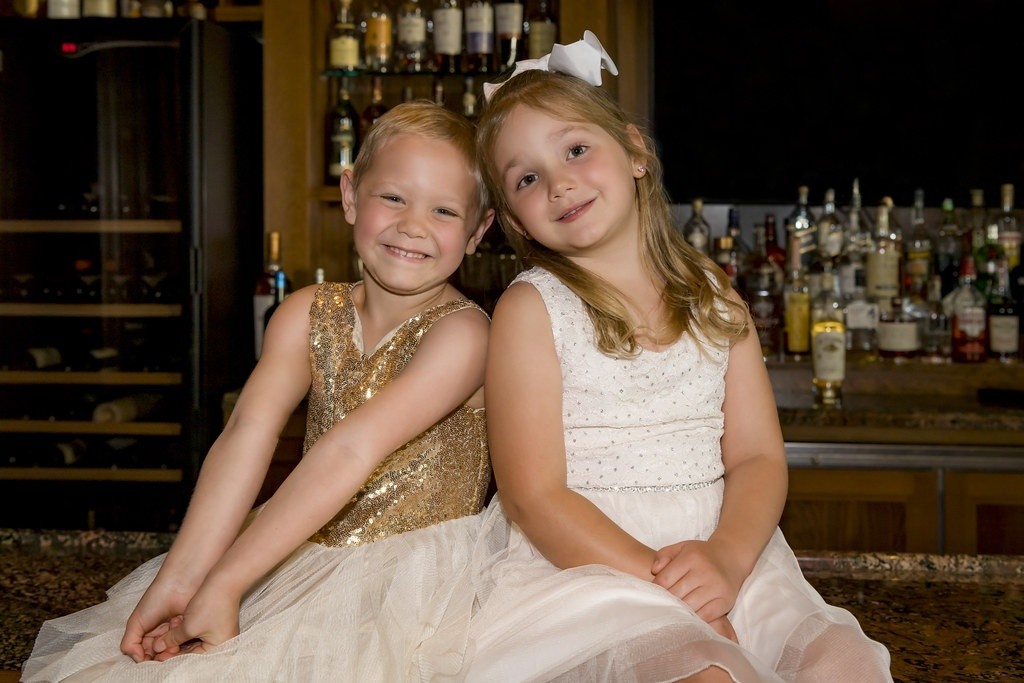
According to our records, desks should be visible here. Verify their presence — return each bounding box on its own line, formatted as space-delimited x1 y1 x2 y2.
223 359 1024 554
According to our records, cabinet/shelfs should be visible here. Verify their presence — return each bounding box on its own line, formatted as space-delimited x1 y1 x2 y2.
0 0 655 531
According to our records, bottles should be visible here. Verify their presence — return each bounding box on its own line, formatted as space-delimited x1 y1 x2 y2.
321 0 558 188
263 268 286 332
315 268 324 285
253 232 284 366
807 260 847 411
683 181 1024 364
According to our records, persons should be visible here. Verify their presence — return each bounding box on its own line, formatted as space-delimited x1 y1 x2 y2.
17 95 491 683
474 29 896 683
